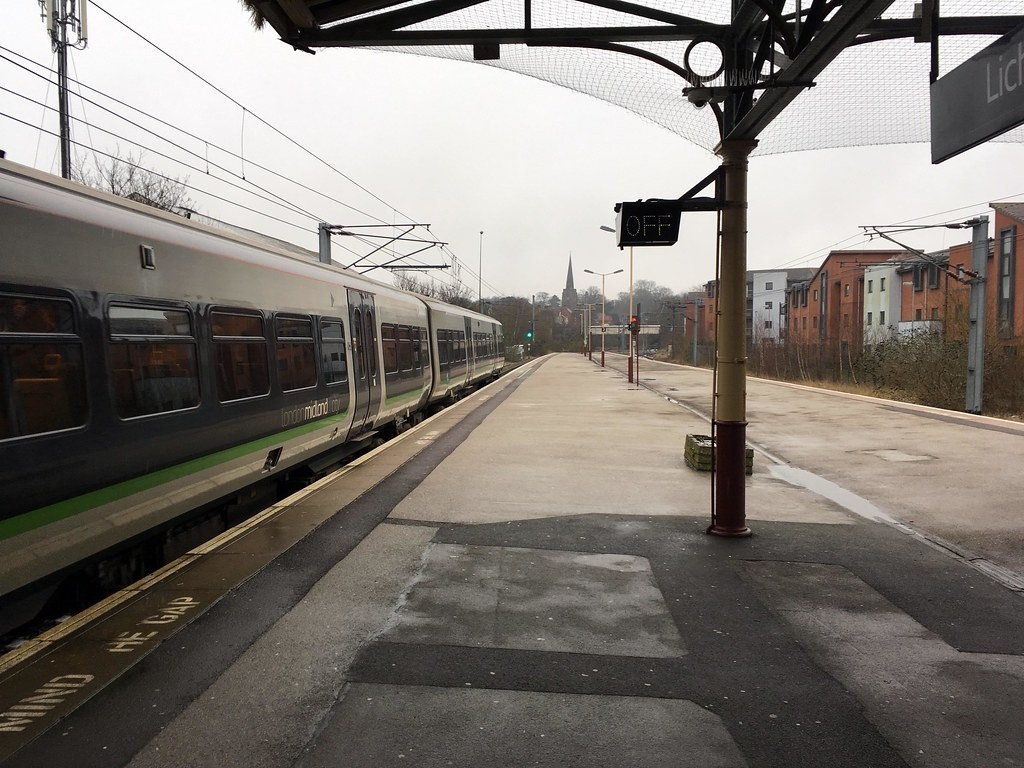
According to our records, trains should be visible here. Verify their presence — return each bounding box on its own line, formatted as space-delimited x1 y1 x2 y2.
0 154 506 605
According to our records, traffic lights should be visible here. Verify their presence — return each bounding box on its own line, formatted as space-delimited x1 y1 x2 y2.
630 315 637 329
527 332 532 339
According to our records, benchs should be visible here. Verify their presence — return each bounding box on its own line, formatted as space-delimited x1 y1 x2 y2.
14 360 315 433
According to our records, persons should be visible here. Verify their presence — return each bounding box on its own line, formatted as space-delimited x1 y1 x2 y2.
0 299 58 379
34 330 305 422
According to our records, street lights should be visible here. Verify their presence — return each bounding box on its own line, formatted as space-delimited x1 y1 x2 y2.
577 302 603 360
574 308 596 356
584 269 624 368
